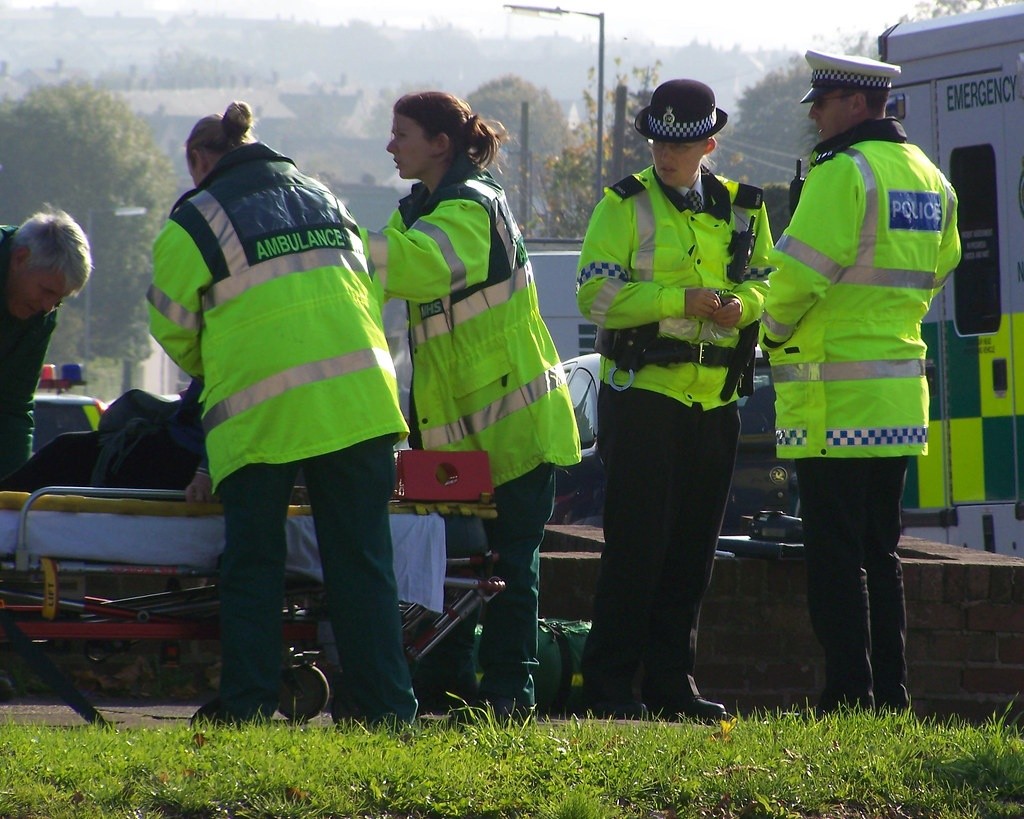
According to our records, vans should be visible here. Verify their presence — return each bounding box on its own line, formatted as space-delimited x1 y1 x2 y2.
383 250 601 448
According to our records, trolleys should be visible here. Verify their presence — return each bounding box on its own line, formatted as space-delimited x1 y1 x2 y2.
0 483 516 727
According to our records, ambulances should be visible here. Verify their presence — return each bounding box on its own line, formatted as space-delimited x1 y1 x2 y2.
872 4 1024 567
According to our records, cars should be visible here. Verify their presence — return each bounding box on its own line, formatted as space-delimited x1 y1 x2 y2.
30 365 111 465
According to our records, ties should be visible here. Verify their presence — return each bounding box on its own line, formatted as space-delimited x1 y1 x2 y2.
686 190 703 214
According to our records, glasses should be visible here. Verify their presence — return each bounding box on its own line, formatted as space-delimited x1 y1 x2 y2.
813 92 855 111
645 138 709 154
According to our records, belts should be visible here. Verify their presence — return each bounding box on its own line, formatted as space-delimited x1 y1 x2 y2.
647 337 770 367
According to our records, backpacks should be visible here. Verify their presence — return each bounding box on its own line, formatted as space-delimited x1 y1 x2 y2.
0 374 205 502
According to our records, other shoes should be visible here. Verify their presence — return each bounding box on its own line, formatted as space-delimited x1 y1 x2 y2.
190 696 271 727
800 703 835 720
584 691 649 719
643 690 727 719
458 692 535 737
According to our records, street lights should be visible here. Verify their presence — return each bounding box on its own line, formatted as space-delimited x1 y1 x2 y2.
500 4 607 226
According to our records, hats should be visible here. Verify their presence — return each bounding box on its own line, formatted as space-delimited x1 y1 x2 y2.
800 49 902 103
635 80 728 143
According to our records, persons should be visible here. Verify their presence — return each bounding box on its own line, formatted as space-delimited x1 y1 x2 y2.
0 377 306 506
0 202 95 478
361 91 581 732
577 79 774 724
145 101 421 728
759 50 961 721
